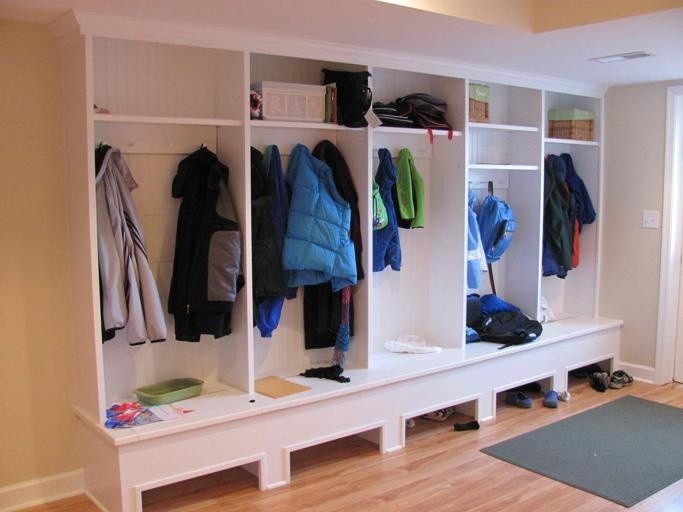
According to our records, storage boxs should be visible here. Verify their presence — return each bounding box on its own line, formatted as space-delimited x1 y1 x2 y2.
253 80 326 120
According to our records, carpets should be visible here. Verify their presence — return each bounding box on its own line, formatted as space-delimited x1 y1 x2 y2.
479 393 683 510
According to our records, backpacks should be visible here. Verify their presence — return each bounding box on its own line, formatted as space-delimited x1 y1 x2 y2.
467 293 542 350
478 181 517 262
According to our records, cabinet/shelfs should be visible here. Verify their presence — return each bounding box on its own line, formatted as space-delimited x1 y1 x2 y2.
47 9 627 511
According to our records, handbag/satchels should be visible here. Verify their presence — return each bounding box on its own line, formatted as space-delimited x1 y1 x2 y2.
321 67 372 127
373 93 450 130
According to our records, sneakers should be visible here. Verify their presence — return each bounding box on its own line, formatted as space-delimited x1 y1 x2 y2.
588 370 633 391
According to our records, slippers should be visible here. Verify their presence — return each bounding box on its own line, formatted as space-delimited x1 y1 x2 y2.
506 391 558 408
